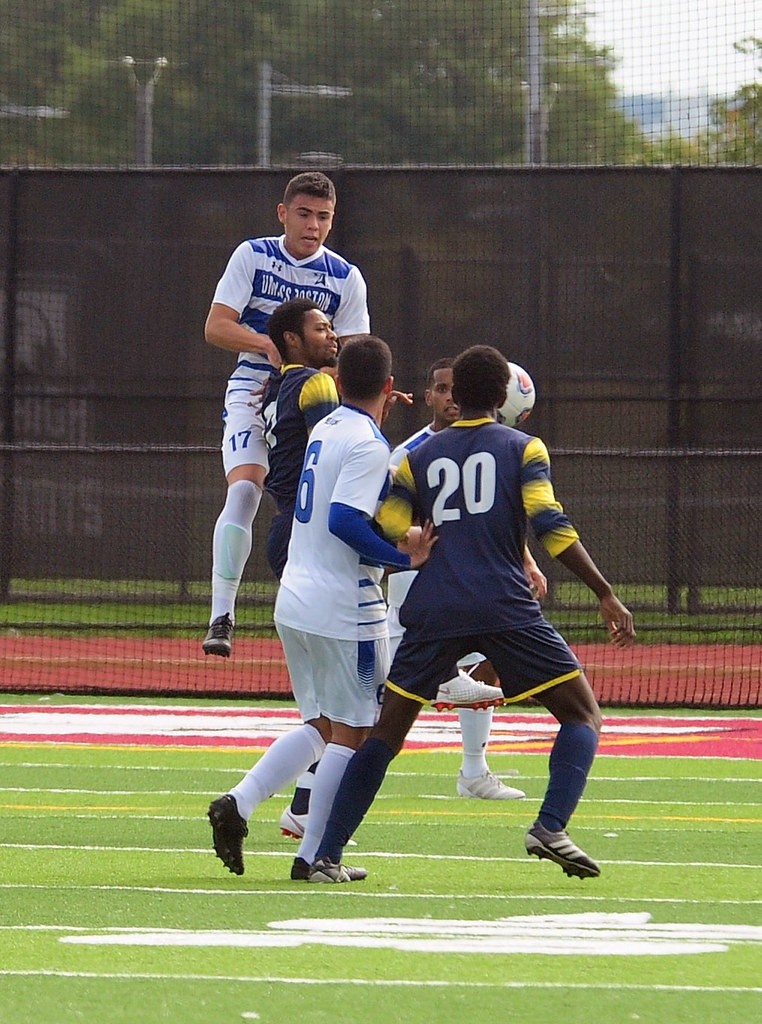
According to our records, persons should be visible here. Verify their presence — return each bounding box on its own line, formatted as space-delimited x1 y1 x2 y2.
201 173 640 886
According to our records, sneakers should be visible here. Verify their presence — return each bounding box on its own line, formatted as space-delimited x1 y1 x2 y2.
207 794 249 875
202 612 234 657
430 668 506 711
525 821 600 880
458 769 526 800
290 858 366 881
307 860 350 884
279 805 356 845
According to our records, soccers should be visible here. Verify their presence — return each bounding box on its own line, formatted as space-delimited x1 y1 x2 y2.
495 361 536 428
